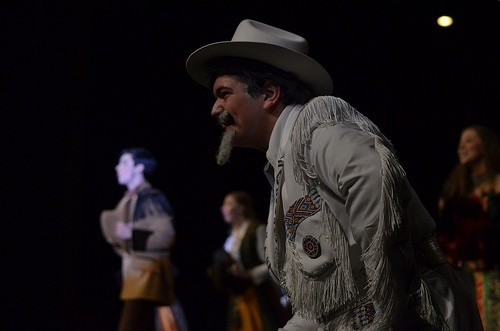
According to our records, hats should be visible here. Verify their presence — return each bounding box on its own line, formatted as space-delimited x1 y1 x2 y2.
186 19 333 97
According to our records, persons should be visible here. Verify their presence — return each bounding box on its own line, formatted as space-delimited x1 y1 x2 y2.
101 147 187 331
187 20 485 331
437 125 500 331
205 191 273 331
